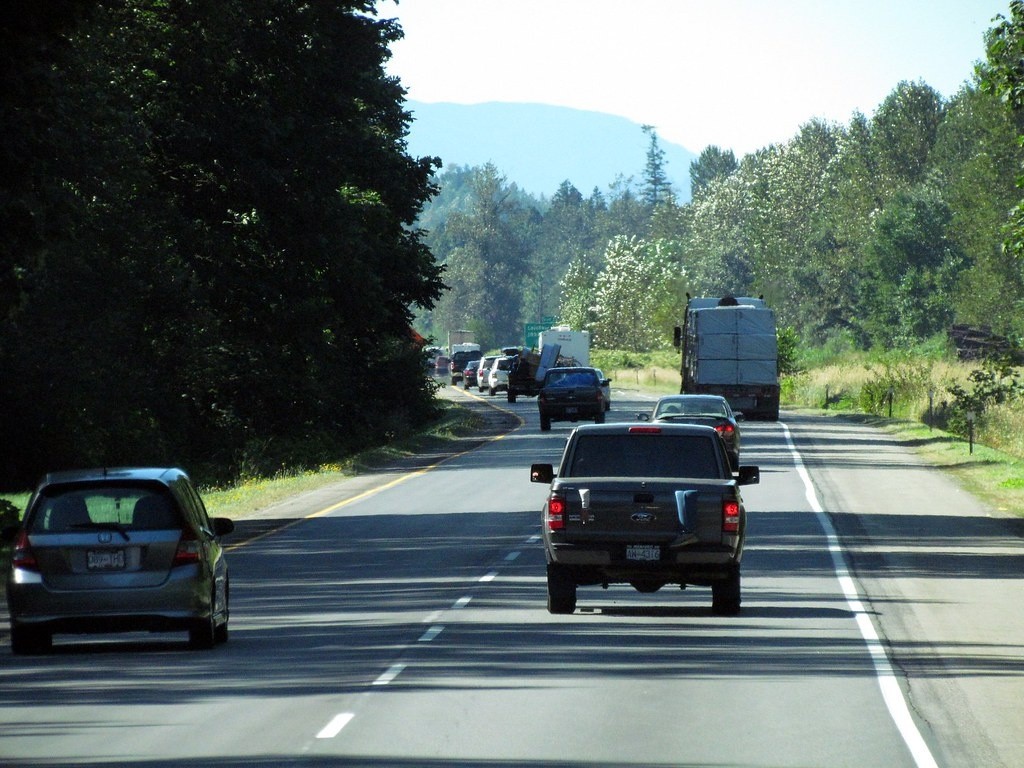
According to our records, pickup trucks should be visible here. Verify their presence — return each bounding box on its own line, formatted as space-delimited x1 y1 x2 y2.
530 421 761 617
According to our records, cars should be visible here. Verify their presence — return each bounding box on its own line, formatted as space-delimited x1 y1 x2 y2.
595 369 612 411
1 467 237 654
635 392 742 473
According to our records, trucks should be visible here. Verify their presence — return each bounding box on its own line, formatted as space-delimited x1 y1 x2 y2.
673 289 780 421
538 329 591 367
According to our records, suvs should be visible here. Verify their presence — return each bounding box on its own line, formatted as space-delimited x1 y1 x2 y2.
537 365 606 432
422 342 575 403
